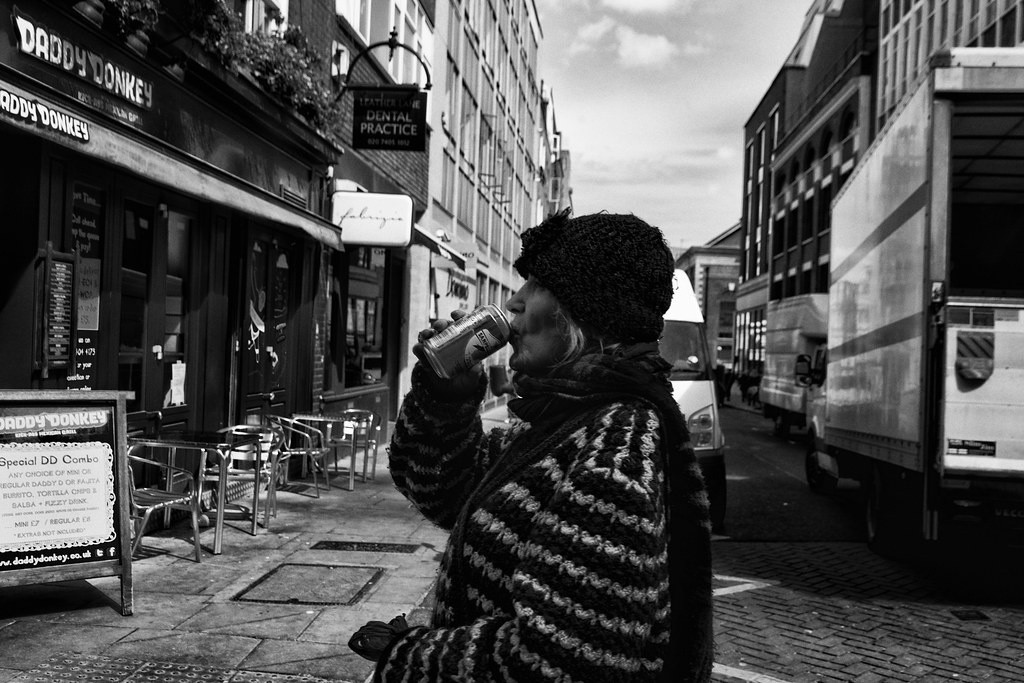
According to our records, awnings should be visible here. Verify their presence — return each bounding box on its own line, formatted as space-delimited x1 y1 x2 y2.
412 223 467 275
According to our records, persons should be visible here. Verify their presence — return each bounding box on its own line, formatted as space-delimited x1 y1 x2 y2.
724 369 762 409
371 214 713 683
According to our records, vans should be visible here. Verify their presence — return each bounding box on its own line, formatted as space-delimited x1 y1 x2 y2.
487 267 735 530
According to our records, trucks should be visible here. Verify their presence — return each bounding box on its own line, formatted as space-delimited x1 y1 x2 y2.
792 43 1023 589
754 293 833 448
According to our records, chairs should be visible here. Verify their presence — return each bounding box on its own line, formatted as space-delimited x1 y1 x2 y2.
124 409 382 564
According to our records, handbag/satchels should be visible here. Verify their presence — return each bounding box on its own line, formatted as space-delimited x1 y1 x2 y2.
748 386 758 394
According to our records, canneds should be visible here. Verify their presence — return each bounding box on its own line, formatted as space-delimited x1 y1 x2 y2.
423 304 512 380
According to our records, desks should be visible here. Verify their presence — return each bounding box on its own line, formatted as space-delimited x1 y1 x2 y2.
285 411 372 491
127 429 263 554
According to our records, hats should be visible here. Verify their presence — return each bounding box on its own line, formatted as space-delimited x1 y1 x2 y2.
513 207 675 345
